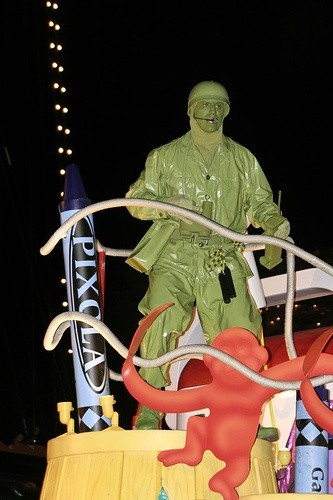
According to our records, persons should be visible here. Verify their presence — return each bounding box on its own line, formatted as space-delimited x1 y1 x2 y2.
125 79 291 443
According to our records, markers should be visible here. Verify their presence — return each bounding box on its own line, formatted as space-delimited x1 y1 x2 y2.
57 161 113 432
293 368 330 494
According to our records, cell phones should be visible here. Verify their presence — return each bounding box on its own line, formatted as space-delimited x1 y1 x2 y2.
259 188 285 269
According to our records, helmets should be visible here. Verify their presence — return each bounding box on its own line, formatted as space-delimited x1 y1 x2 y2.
187 81 230 116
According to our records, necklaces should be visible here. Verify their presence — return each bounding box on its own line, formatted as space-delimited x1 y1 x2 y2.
192 138 218 180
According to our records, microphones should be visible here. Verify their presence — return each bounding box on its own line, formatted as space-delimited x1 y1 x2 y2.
194 117 218 124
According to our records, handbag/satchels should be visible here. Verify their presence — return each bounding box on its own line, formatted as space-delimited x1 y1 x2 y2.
127 220 177 273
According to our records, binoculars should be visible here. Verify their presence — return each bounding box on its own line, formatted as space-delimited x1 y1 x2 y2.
179 201 213 240
